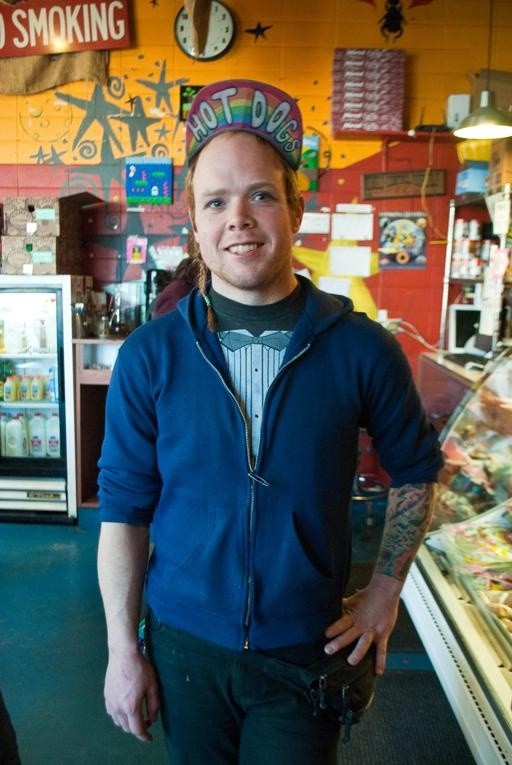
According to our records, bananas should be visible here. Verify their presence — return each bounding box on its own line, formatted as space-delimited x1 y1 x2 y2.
290 217 427 277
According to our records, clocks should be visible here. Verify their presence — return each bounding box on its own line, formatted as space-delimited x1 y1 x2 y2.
174 1 235 61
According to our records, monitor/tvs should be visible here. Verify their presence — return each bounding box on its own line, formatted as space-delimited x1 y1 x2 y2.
448 302 512 354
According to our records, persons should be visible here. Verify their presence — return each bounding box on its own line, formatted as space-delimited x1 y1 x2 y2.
97 79 446 765
153 257 198 317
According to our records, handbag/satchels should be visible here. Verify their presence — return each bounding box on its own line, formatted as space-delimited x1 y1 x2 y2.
314 637 373 721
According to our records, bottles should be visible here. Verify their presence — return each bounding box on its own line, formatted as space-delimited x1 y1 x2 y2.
2 358 57 404
1 412 60 459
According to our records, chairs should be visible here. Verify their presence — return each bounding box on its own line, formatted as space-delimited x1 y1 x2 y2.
350 428 389 525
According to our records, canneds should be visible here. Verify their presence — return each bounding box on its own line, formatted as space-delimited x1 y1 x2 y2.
451 217 500 279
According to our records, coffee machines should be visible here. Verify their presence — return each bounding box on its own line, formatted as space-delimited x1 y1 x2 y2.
134 270 167 330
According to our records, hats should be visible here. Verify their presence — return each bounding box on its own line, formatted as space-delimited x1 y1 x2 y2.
186 78 303 168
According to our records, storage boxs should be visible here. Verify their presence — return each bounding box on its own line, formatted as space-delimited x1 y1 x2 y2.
1 238 85 276
3 191 106 239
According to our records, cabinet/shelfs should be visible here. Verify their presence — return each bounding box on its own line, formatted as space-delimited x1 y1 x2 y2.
71 337 131 509
438 182 512 360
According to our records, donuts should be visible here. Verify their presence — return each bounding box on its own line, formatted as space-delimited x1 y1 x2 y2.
395 252 409 265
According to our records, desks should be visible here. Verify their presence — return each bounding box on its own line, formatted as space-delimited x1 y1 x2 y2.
418 351 488 424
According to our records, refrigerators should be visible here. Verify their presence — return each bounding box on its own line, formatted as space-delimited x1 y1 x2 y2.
1 272 94 526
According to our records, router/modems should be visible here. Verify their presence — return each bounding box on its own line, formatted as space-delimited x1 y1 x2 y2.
415 106 452 133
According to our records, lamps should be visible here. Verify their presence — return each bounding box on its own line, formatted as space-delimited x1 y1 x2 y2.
452 1 512 139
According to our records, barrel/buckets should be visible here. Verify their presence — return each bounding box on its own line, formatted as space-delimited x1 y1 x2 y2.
0 411 60 460
